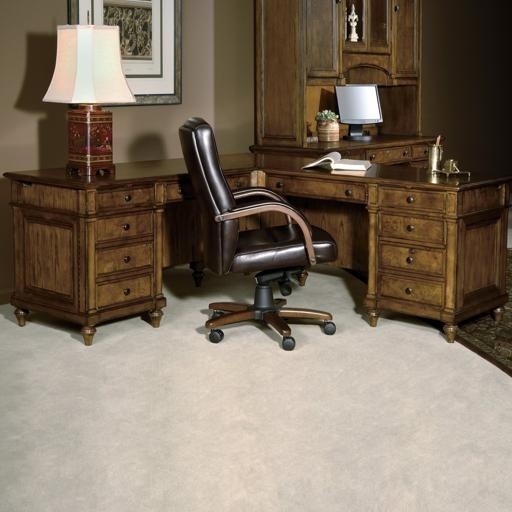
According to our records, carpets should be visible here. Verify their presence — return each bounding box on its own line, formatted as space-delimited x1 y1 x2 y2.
453 248 512 377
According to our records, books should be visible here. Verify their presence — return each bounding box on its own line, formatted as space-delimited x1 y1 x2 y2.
299 147 375 175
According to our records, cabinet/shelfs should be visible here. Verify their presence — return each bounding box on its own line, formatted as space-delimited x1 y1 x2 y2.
254 0 445 270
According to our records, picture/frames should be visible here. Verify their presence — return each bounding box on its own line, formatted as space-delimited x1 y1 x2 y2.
67 0 184 106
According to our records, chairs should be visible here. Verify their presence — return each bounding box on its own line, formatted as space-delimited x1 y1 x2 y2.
175 114 339 351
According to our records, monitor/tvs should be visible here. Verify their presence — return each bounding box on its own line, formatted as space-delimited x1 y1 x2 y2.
333 83 384 141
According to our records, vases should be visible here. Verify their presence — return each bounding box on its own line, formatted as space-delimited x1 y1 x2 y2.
316 121 340 142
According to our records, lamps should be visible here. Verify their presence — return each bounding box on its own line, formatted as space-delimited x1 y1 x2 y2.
42 11 139 177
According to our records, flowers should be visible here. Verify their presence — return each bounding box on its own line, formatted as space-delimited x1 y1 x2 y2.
315 109 340 122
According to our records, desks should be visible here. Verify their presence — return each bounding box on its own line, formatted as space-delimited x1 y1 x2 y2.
2 154 509 347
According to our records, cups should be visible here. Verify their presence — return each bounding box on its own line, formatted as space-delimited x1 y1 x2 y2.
428 144 444 173
